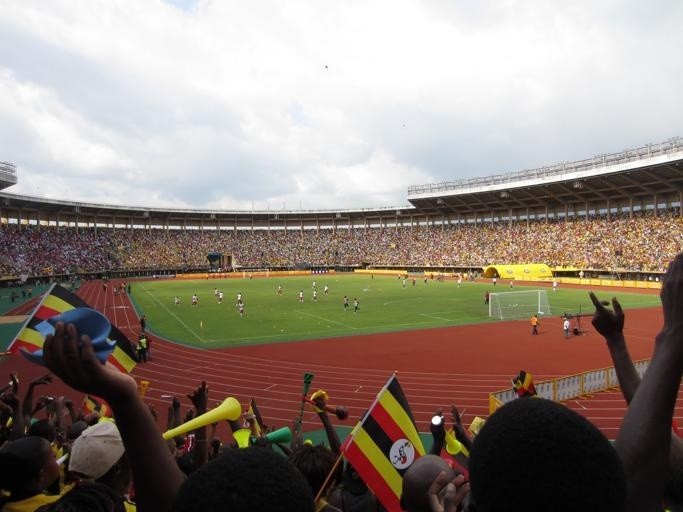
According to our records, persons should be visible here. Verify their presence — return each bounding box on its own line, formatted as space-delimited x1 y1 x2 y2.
1 367 683 510
530 314 541 336
41 319 187 510
125 312 153 363
273 286 361 315
612 251 682 511
100 278 132 299
1 209 682 289
585 288 682 512
563 316 570 338
171 287 245 320
7 285 49 305
483 290 490 305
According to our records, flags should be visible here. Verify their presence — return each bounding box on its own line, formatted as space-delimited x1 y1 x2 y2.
5 282 141 375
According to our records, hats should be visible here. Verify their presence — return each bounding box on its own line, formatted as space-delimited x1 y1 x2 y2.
67 419 128 483
